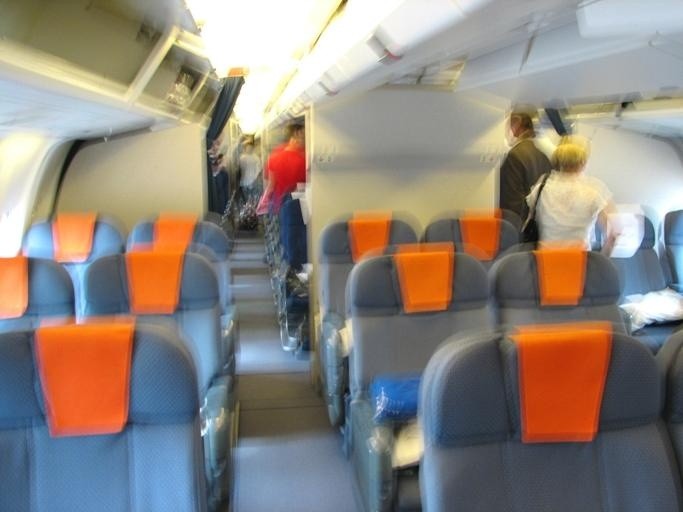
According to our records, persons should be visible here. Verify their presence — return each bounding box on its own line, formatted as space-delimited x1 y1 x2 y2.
499 111 623 256
208 139 228 209
237 138 262 201
257 125 305 215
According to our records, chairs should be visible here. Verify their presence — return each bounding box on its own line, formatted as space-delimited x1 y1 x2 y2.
1 161 683 511
